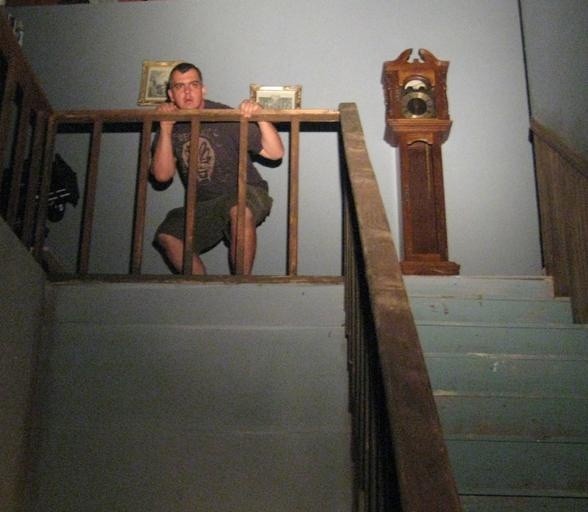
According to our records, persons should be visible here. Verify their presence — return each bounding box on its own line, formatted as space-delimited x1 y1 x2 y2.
149 63 285 275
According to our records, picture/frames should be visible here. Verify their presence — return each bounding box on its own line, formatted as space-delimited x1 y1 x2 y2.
136 60 181 106
249 83 303 111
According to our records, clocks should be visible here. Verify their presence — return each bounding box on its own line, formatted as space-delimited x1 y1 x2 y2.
381 48 461 276
